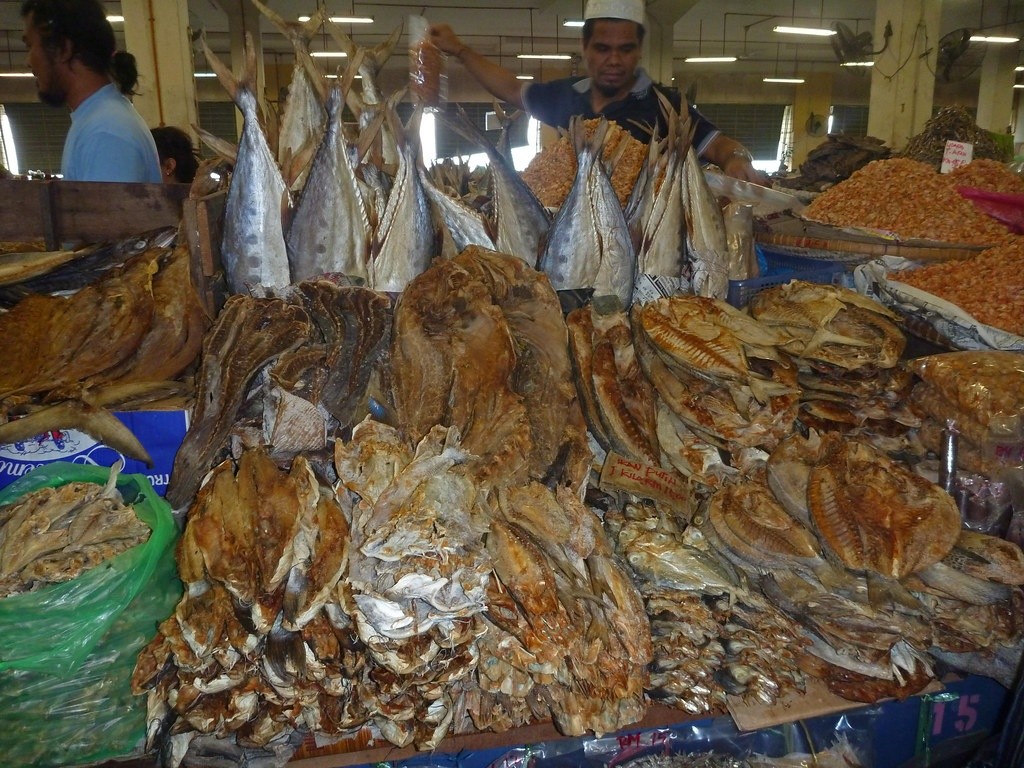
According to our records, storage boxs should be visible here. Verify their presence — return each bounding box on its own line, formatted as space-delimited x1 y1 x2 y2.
0 408 192 497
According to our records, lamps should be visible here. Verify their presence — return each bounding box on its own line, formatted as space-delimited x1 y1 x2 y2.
562 0 585 27
516 8 573 60
840 21 875 67
309 8 348 58
321 35 362 79
297 0 374 24
684 19 738 62
960 0 1020 43
515 60 534 80
772 0 837 37
762 42 805 83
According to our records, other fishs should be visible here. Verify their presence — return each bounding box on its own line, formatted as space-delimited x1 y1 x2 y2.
187 0 757 316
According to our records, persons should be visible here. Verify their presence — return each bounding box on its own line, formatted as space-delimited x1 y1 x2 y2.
418 0 773 195
150 126 199 184
20 0 163 184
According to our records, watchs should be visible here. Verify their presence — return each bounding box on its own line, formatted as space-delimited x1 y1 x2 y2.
722 147 753 169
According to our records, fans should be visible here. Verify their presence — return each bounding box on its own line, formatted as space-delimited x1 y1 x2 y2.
805 112 828 137
829 20 893 77
918 27 988 84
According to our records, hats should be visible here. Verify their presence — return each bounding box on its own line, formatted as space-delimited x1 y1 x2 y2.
584 0 646 33
